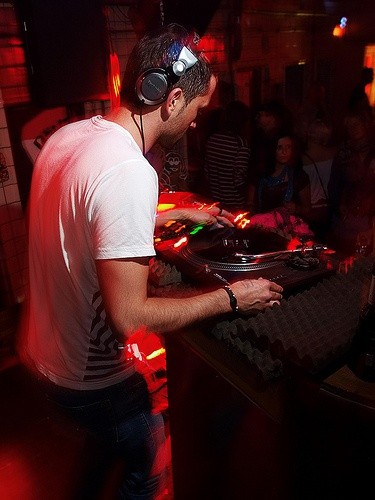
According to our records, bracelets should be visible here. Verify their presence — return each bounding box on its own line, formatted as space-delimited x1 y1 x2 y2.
221 285 240 313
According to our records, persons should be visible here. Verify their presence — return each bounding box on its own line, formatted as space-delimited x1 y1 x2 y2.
34 26 281 490
186 64 373 272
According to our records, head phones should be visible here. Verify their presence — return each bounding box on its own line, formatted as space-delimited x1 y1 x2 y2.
135 46 198 105
